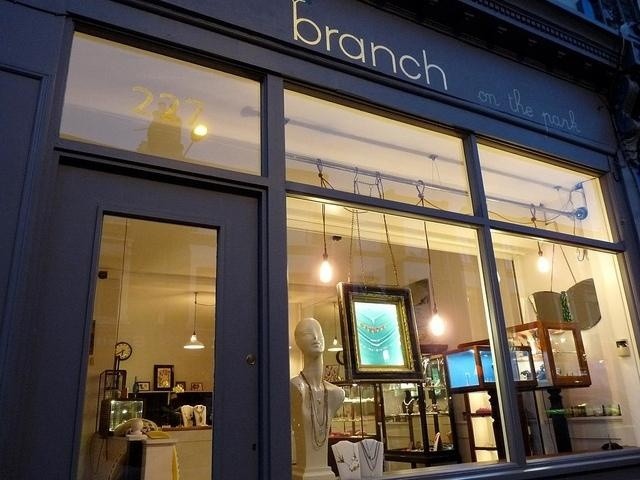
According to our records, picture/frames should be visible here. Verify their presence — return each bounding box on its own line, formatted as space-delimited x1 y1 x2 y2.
338 282 425 385
137 363 203 391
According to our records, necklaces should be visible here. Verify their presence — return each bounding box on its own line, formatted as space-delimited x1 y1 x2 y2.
360 440 380 471
403 398 414 413
333 441 360 473
300 369 330 449
183 407 193 426
356 303 404 366
195 406 206 423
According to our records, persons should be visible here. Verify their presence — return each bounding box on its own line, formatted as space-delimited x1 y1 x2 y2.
290 318 346 468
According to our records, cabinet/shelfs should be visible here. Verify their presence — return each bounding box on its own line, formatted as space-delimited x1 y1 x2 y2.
330 344 499 477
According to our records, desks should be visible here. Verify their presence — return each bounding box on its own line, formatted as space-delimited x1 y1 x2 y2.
94 429 178 480
127 387 214 424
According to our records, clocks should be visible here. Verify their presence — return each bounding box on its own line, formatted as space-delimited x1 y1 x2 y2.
115 340 132 363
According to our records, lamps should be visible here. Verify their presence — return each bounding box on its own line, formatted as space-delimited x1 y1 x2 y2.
414 181 448 338
182 290 206 352
315 161 334 284
529 204 550 275
